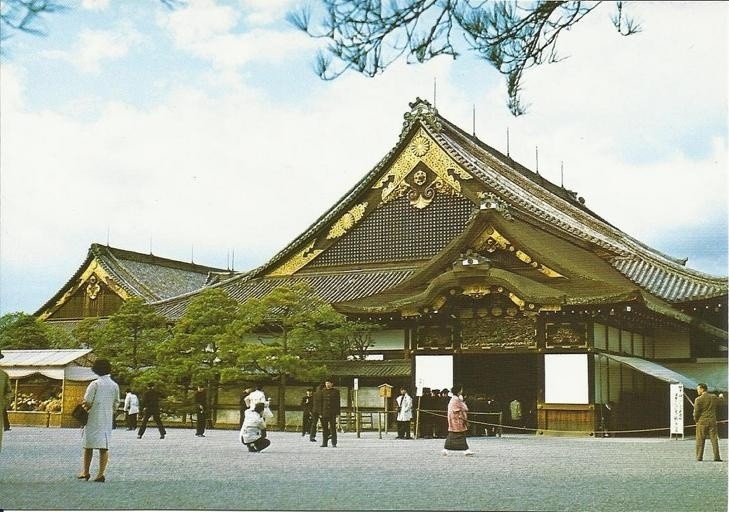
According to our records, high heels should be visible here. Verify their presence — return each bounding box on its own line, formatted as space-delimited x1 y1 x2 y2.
76 473 91 481
93 475 106 482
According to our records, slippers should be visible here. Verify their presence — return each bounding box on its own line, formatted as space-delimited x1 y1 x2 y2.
464 449 476 455
441 450 451 457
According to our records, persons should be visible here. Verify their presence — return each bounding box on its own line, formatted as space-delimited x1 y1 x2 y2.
75 357 121 484
122 385 141 431
300 388 317 437
443 382 472 451
394 384 415 439
602 401 614 437
456 395 469 420
690 381 727 464
193 382 209 437
239 382 275 453
2 404 13 431
309 383 332 442
419 386 523 440
317 379 341 447
0 352 15 456
137 381 169 438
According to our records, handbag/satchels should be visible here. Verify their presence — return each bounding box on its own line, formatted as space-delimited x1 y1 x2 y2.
263 407 275 419
70 404 89 425
396 406 402 412
